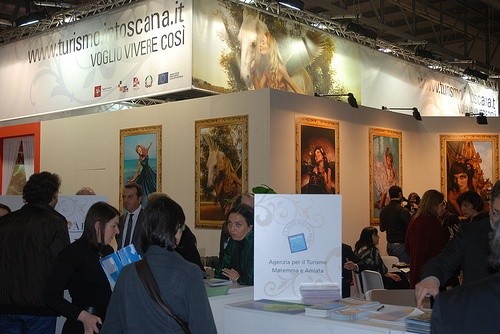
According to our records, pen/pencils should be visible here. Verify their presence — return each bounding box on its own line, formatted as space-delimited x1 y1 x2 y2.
376 305 384 312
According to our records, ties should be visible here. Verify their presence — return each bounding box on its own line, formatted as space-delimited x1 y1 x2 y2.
123 214 134 247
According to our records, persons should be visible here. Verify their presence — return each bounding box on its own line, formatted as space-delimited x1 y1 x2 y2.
353 226 411 290
1 170 71 333
45 200 122 333
142 191 205 278
213 153 243 209
251 30 304 94
113 183 149 251
374 144 399 210
0 203 11 219
99 199 217 333
380 179 500 334
127 141 157 193
306 145 332 193
214 204 255 286
448 162 486 218
341 242 367 299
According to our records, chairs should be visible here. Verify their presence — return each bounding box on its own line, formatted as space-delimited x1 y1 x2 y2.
362 269 385 302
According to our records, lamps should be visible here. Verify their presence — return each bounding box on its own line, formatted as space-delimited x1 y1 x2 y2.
382 106 423 122
465 112 488 125
313 91 359 108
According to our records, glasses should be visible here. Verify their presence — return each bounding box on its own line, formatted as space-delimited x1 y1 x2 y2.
490 202 500 217
176 222 185 233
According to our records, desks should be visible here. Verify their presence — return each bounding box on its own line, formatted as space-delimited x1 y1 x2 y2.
203 276 432 334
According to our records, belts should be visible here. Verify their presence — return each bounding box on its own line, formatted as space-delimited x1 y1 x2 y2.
385 239 407 244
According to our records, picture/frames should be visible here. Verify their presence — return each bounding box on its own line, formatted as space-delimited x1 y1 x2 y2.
117 124 162 217
193 114 250 231
294 116 341 195
438 133 500 221
367 127 404 228
